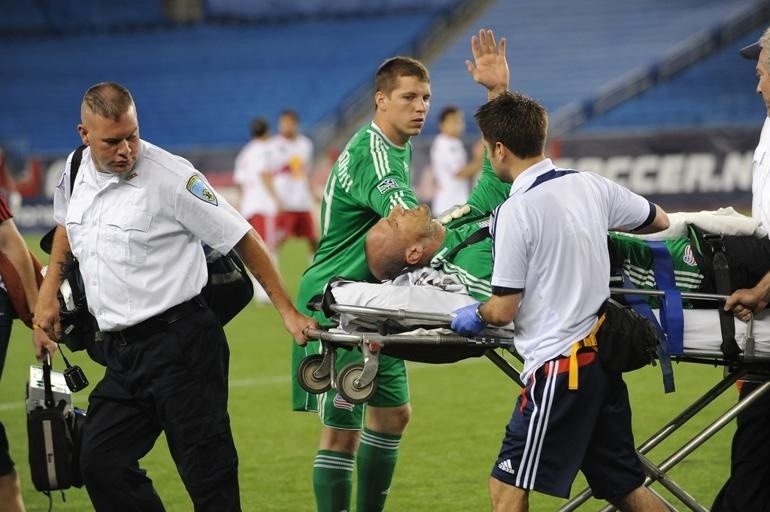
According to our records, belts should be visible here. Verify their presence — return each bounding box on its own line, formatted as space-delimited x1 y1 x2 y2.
105 300 209 348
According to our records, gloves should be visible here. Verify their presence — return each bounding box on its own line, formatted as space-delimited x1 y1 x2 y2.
451 302 486 339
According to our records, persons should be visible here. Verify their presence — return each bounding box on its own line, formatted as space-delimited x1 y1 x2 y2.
364 29 770 305
291 56 431 511
0 198 60 511
429 107 485 217
448 92 669 511
232 110 320 302
710 28 770 512
35 84 316 510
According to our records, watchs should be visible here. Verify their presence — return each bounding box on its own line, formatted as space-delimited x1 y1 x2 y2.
475 303 488 324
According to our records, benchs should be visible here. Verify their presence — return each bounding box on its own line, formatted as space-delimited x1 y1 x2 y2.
0 0 770 153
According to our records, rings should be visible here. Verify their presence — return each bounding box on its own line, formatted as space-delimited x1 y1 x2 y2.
32 325 40 328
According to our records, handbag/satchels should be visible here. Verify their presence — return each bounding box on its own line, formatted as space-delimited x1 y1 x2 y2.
39 215 254 367
28 403 87 492
596 300 659 372
